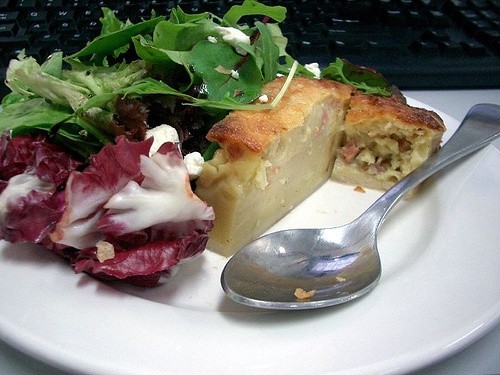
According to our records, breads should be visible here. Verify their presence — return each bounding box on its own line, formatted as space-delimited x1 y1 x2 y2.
195 72 349 256
334 91 445 196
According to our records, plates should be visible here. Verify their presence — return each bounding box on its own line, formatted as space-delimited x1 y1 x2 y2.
0 92 499 374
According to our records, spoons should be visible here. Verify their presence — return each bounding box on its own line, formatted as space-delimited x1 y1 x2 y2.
217 101 500 312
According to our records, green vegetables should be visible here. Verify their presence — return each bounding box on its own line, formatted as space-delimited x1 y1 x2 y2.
0 1 290 128
